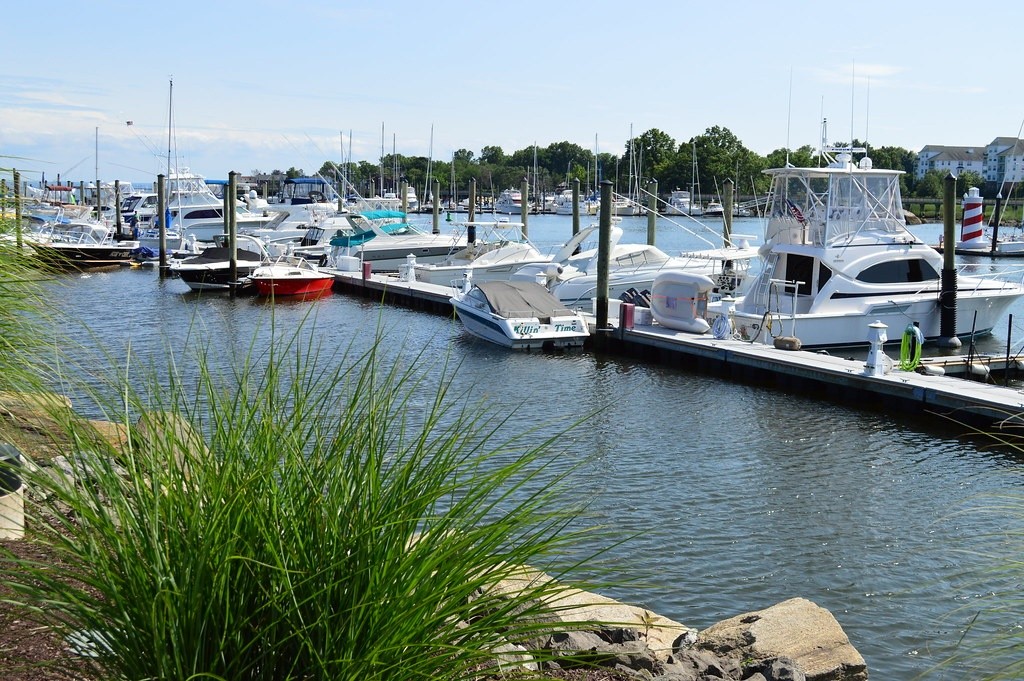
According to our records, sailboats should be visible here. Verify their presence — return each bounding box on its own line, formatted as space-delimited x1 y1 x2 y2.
533 122 648 215
329 121 449 213
666 140 703 214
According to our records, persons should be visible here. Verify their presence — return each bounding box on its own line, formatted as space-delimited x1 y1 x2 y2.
129 211 141 237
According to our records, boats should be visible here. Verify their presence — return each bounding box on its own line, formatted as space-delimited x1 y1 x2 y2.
707 198 725 213
448 280 590 348
509 216 761 308
707 148 1021 344
164 173 404 250
402 220 568 285
169 234 274 290
494 186 531 215
248 253 336 293
23 173 159 264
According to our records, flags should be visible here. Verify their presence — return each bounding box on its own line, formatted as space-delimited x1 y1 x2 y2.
785 197 806 223
